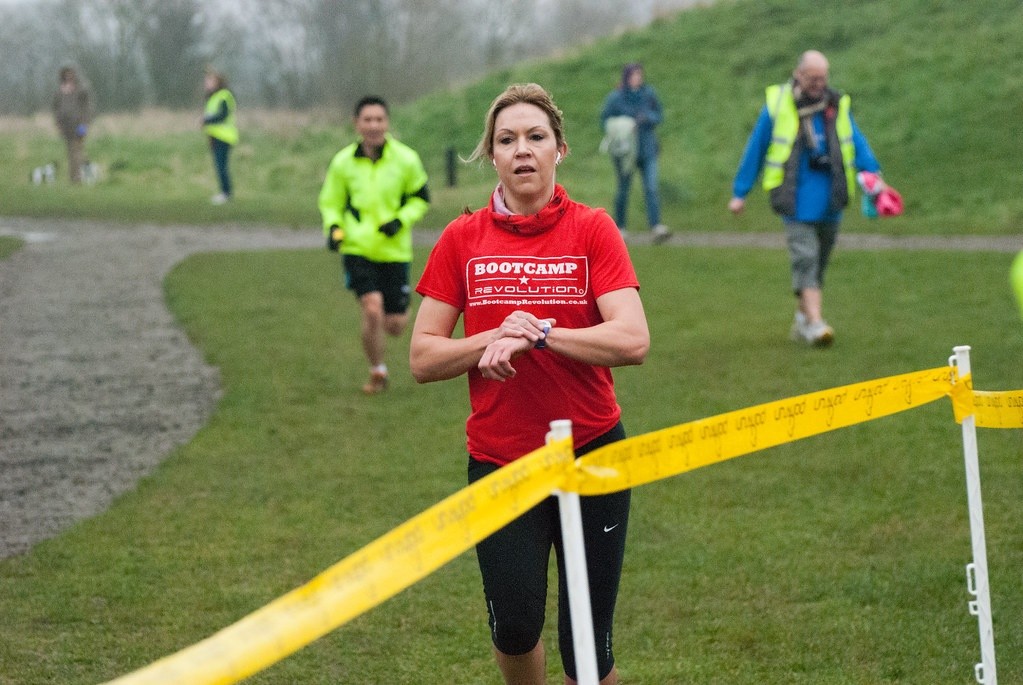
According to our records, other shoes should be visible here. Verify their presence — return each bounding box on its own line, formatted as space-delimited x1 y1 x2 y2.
650 225 673 247
804 323 834 346
619 226 631 242
362 373 388 395
789 310 806 335
212 190 230 205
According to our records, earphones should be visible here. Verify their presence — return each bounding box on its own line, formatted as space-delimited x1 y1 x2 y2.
555 151 561 164
492 158 496 166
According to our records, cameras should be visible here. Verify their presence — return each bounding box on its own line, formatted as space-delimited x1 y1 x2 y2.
810 155 832 172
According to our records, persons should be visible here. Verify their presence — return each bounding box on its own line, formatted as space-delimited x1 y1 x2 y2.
407 80 649 685
727 50 904 348
316 95 432 396
198 69 239 206
50 61 96 185
599 64 672 243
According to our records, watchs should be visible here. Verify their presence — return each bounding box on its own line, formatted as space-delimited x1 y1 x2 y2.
535 320 552 349
